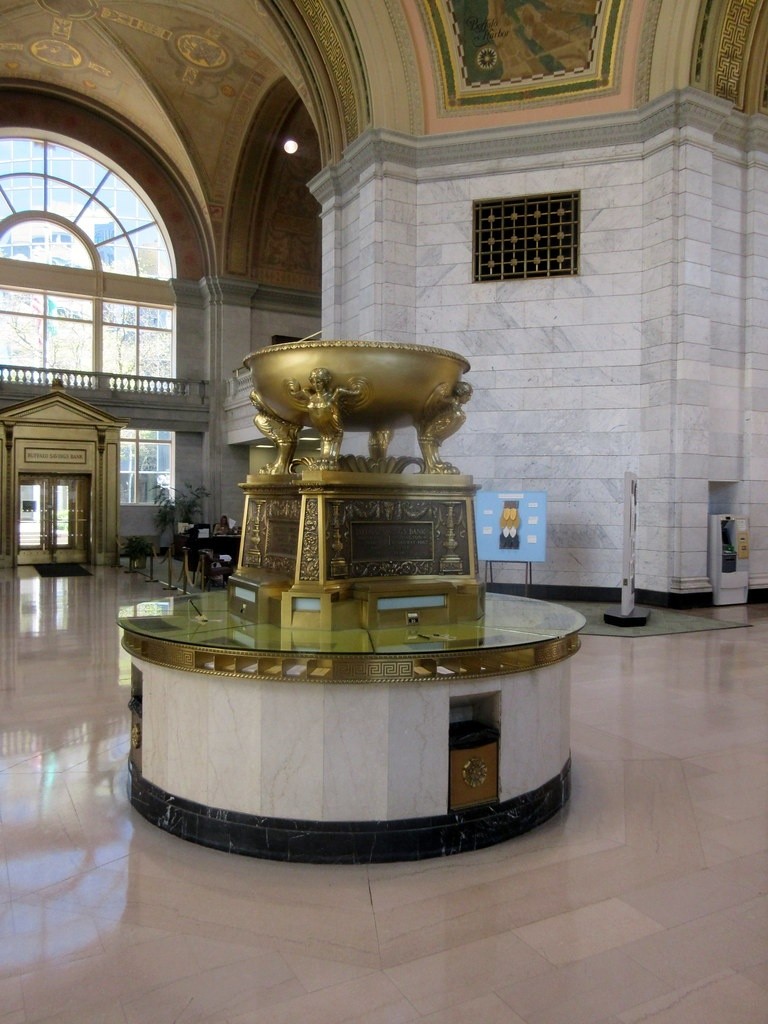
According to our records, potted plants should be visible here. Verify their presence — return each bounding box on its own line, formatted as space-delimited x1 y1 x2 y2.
122 536 154 570
148 481 212 557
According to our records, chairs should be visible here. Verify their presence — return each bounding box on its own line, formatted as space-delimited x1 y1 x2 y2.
199 548 234 591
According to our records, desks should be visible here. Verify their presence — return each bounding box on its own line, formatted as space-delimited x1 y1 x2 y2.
175 533 241 567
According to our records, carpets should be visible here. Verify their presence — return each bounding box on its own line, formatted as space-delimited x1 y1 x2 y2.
553 602 754 638
33 563 92 577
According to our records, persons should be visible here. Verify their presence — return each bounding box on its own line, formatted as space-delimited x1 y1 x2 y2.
424 382 473 468
213 515 229 535
290 368 361 456
250 392 280 468
